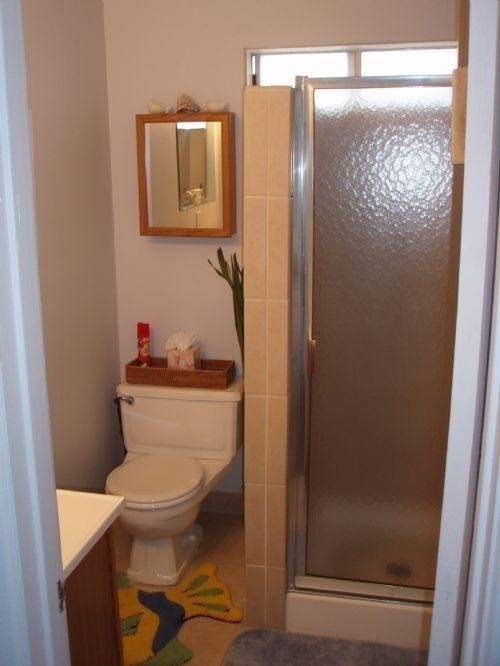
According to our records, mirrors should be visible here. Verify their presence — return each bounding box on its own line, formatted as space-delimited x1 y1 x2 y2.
176 122 216 212
135 112 237 238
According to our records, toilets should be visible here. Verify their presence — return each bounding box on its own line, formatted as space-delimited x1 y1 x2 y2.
104 451 234 589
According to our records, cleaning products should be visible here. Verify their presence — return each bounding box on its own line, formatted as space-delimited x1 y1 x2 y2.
137 320 154 365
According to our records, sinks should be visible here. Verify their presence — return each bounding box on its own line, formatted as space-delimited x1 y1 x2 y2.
55 488 125 580
